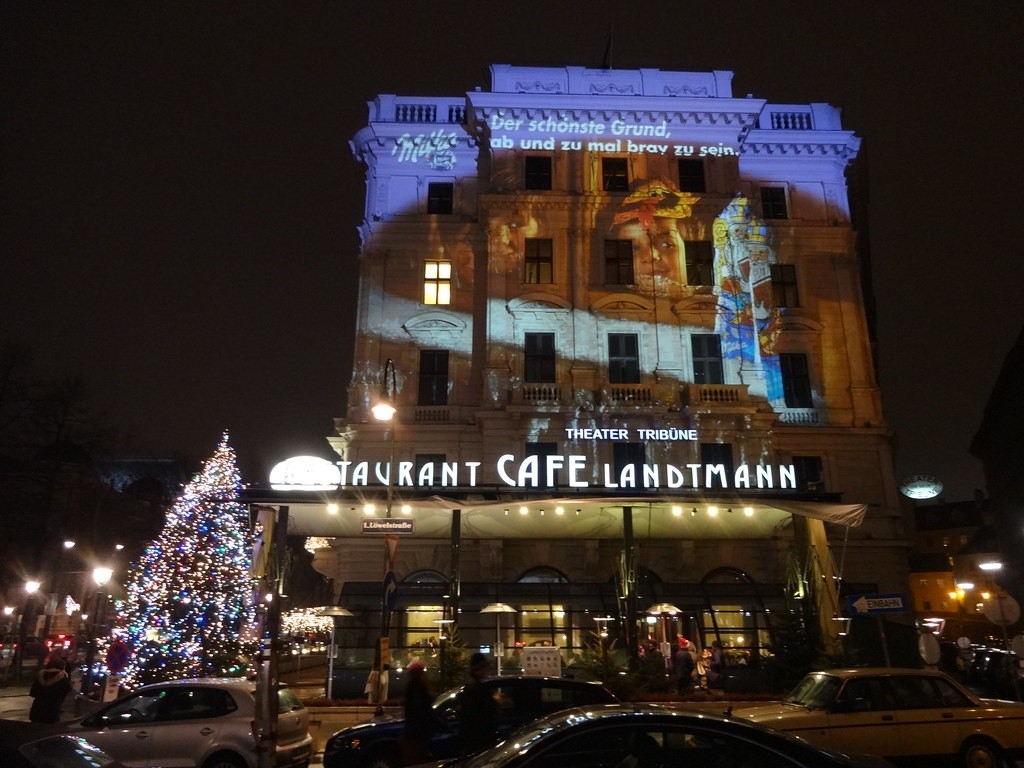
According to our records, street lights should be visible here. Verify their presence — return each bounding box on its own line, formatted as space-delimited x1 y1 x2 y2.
83 564 115 675
369 357 399 716
315 604 354 699
979 559 1022 702
478 601 519 693
14 580 40 685
43 536 78 639
102 541 125 614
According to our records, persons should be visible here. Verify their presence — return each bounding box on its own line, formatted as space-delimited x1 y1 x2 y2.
402 651 501 768
637 634 733 698
608 175 692 292
27 655 78 726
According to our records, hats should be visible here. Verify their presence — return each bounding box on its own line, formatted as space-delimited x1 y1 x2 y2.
958 636 972 649
409 663 424 679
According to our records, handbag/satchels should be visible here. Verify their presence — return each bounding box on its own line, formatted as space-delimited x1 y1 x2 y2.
30 686 41 698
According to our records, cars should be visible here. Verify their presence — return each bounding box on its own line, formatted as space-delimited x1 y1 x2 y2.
47 676 316 768
726 666 1024 768
0 714 130 767
322 671 622 768
46 633 75 651
405 699 894 768
0 631 49 660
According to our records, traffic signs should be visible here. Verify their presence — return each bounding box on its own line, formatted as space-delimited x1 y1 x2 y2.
850 592 909 614
